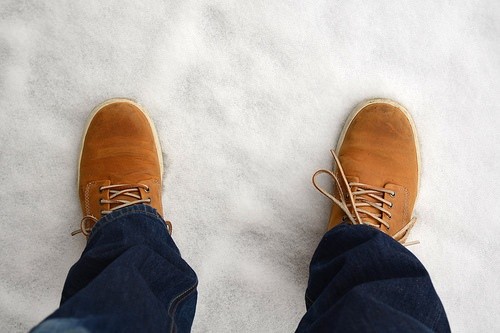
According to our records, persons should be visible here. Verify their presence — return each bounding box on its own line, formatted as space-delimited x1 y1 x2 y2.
27 99 451 333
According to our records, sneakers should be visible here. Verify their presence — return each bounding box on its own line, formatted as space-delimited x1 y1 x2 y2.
312 99 420 249
70 98 172 239
